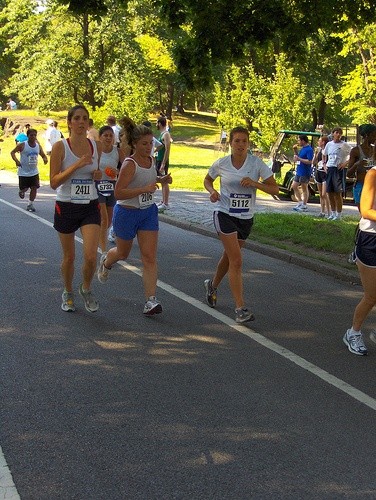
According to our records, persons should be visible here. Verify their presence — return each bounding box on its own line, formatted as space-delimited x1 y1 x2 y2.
312 136 330 218
87 119 99 141
348 124 376 214
322 128 352 221
343 165 376 355
98 126 126 263
13 124 31 146
108 127 135 244
120 117 136 131
204 127 279 323
106 115 122 147
221 125 227 146
10 98 16 109
155 117 171 211
45 120 64 155
50 105 103 313
97 125 173 313
11 129 48 212
142 121 163 157
292 135 313 212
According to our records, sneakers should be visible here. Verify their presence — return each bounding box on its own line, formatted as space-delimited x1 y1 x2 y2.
294 203 302 209
204 279 216 307
19 191 25 199
143 295 162 314
332 216 341 220
298 204 307 210
343 329 368 355
234 307 255 322
61 291 76 312
79 284 100 312
327 215 334 219
97 252 112 285
27 204 35 212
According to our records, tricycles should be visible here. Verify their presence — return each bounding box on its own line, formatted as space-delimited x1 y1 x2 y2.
265 123 362 207
